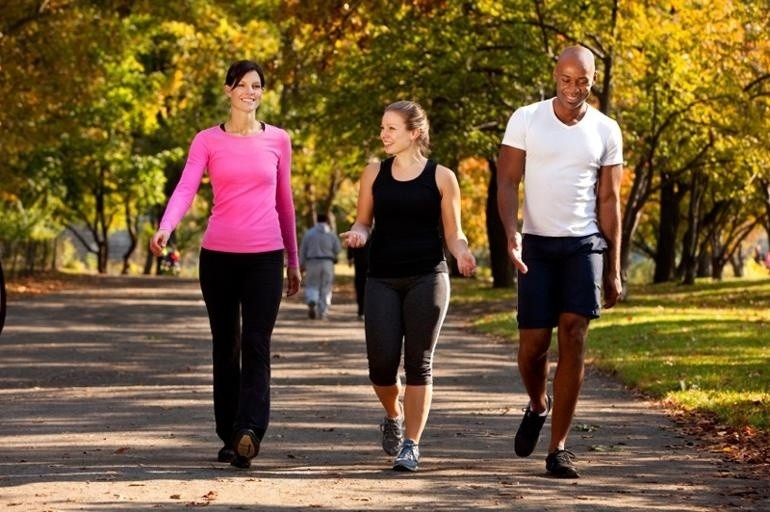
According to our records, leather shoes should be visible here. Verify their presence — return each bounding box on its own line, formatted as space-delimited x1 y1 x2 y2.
217 444 235 462
230 426 261 469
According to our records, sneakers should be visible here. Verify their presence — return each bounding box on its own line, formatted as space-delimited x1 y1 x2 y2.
544 448 581 477
306 300 316 320
391 438 421 472
378 401 405 458
512 392 553 458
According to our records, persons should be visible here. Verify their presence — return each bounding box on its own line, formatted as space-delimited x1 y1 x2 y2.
167 243 181 276
300 210 343 317
753 243 763 264
335 98 477 471
764 248 770 273
495 45 625 481
347 216 374 321
148 59 303 468
